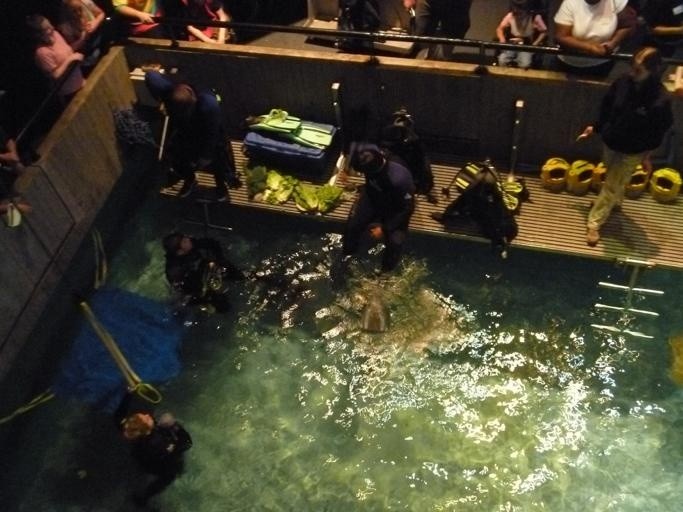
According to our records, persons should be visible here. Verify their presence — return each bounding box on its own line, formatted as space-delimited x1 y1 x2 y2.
49 0 107 65
1 122 25 176
492 2 549 70
552 0 638 80
23 13 88 110
332 0 383 55
142 68 247 199
175 2 239 52
160 222 250 314
124 411 194 481
109 1 166 41
325 141 417 288
399 1 476 64
571 46 674 249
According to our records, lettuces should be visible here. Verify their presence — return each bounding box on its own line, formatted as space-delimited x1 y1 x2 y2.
265 169 343 213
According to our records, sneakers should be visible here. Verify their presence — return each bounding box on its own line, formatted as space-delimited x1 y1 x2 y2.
175 174 231 204
586 225 602 248
